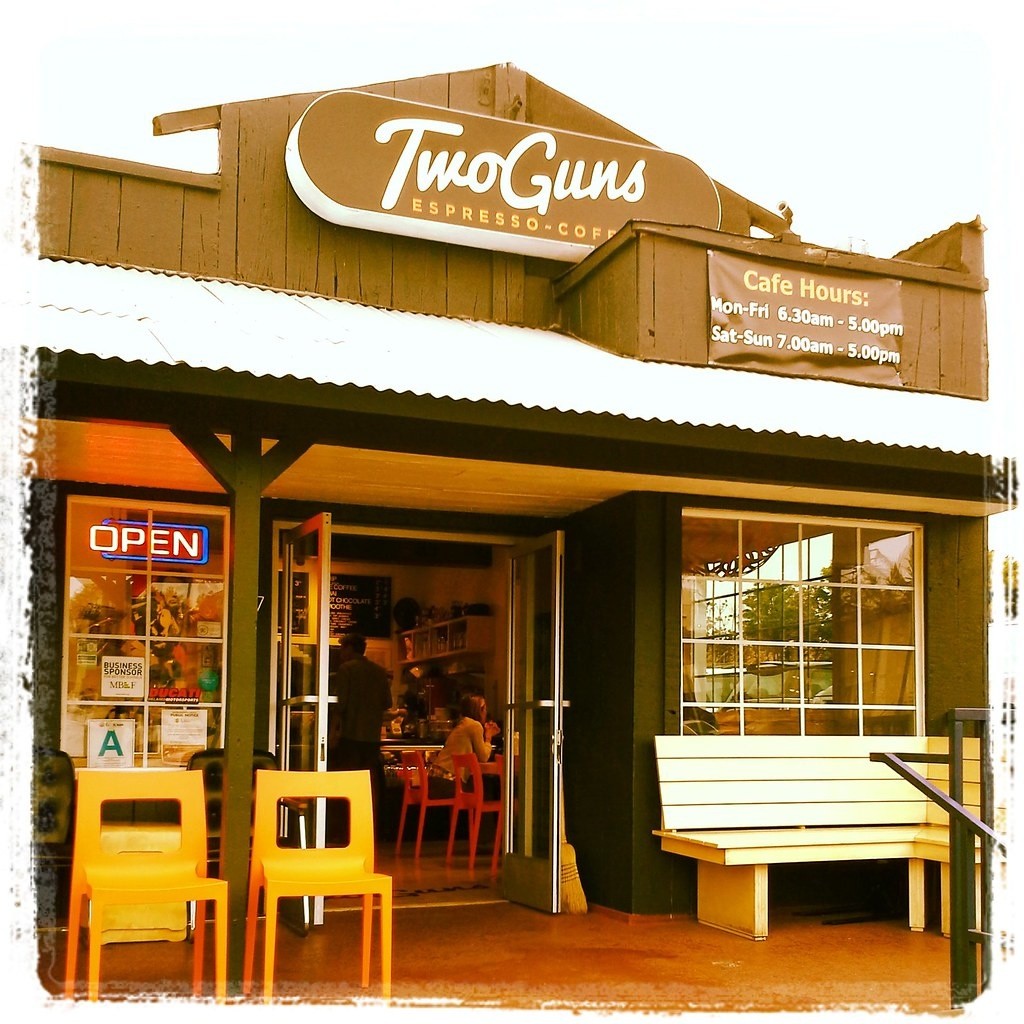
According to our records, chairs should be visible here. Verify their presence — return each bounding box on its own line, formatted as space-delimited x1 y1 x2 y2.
244 769 393 998
64 771 227 996
395 750 520 873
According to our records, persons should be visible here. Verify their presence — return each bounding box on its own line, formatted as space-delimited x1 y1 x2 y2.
424 694 502 835
321 636 392 841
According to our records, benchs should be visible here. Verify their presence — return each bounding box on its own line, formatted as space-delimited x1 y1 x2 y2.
653 736 981 940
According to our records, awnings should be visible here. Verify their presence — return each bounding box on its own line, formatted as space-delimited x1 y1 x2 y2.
0 246 1017 512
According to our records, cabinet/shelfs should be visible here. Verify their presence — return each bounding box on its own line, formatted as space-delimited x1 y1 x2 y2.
397 616 494 679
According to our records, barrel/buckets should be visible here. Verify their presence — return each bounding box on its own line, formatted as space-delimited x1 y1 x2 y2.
435 708 450 720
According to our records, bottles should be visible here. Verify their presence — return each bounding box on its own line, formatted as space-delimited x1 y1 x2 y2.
135 715 143 753
148 720 155 752
414 600 465 656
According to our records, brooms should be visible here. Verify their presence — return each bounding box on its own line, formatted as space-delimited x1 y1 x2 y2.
559 785 590 917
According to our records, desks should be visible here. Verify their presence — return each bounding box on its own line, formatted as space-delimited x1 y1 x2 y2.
470 762 500 775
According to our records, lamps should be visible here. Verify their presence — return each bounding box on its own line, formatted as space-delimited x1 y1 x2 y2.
70 577 84 597
